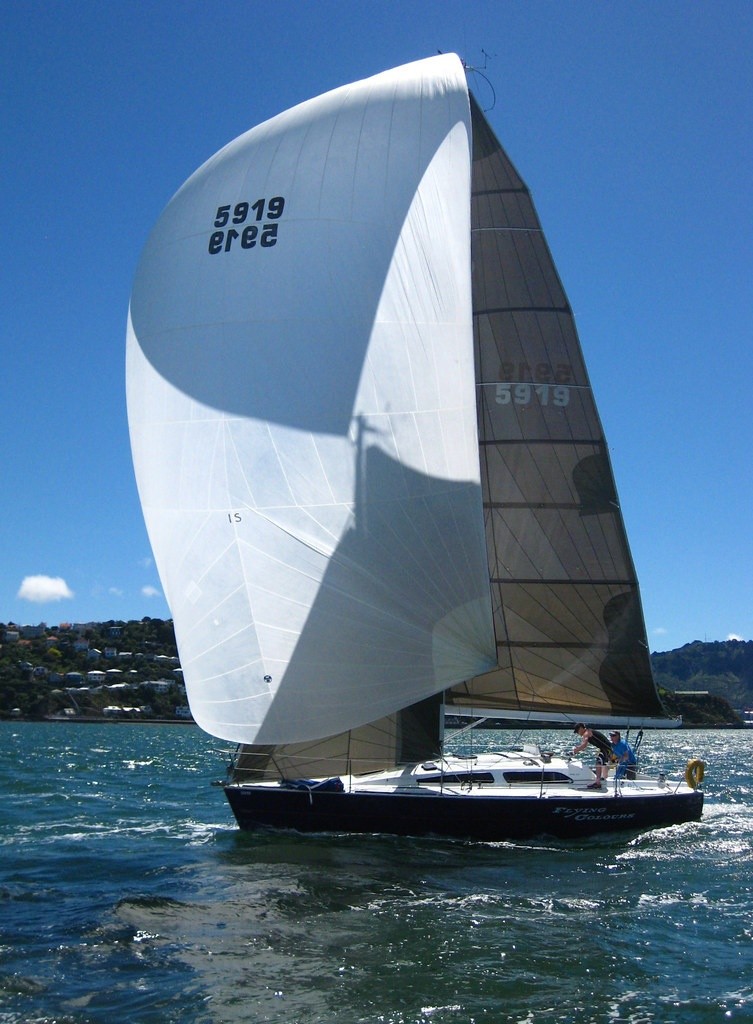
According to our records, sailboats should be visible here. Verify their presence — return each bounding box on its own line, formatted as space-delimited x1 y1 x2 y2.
125 49 709 844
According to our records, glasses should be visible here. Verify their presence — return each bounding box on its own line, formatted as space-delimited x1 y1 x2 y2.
610 735 616 737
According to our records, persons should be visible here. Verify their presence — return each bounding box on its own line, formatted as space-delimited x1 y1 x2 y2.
573 723 612 790
609 731 637 780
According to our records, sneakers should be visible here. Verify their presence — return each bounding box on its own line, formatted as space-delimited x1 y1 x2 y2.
587 783 602 789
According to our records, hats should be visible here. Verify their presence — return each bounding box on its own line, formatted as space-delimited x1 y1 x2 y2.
609 731 620 736
574 723 585 733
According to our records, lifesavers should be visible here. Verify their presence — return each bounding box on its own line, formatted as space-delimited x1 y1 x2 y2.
685 758 704 789
